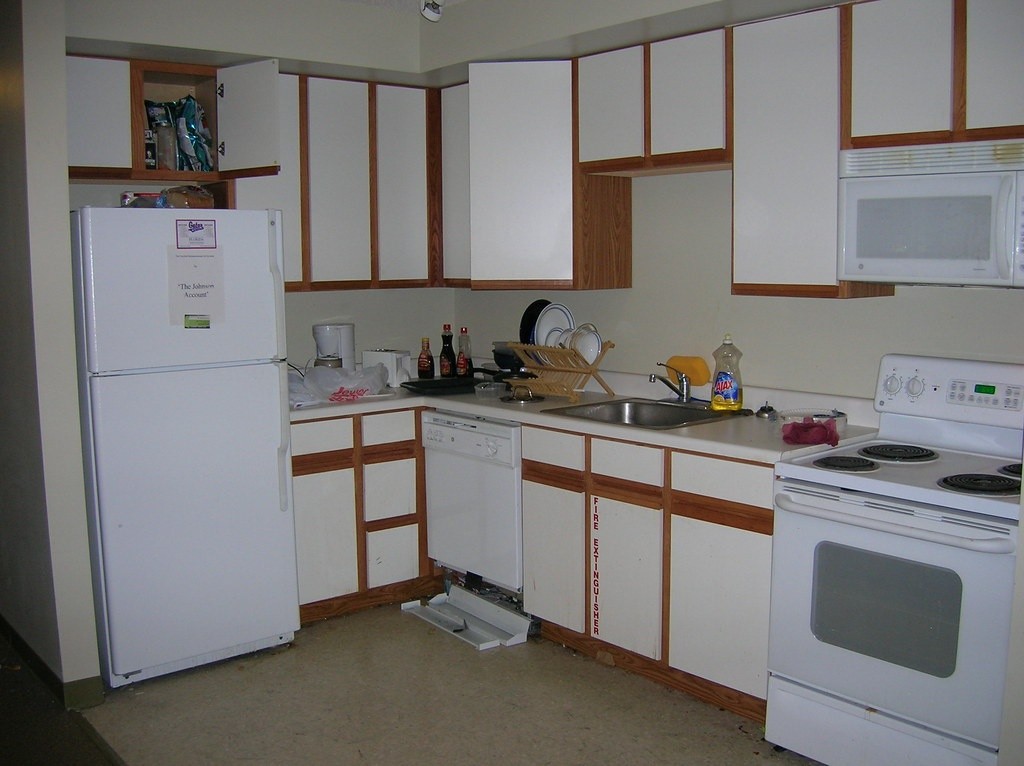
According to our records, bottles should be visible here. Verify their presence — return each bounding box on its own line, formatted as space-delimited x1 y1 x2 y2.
457 327 472 377
440 324 456 378
418 337 434 379
711 334 743 410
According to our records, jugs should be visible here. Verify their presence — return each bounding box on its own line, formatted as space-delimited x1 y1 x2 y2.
314 355 341 369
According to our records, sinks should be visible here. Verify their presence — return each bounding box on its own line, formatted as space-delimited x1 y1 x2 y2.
543 397 732 430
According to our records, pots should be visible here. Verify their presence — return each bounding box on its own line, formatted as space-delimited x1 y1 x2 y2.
468 366 537 389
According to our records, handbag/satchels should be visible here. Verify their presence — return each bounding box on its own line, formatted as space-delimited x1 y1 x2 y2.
303 362 389 402
287 371 321 409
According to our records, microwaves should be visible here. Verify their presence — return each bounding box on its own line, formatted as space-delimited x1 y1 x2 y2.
835 138 1024 289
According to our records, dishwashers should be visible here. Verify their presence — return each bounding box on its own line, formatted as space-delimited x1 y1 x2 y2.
421 407 523 593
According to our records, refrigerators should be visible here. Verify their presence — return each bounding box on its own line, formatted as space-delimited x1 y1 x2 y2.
71 205 300 692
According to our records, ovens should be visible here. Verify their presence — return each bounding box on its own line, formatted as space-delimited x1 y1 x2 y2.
764 479 1018 766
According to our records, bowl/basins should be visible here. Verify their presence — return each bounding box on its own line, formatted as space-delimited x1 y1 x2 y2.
474 382 506 401
492 342 523 371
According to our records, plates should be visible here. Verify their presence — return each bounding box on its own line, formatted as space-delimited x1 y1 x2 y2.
520 299 601 366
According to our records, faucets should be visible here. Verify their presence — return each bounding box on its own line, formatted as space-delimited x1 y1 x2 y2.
649 361 711 410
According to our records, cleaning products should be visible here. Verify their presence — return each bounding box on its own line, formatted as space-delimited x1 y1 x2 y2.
712 333 745 411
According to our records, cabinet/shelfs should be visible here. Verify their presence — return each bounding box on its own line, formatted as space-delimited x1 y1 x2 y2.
518 426 775 722
290 409 428 625
66 1 1024 298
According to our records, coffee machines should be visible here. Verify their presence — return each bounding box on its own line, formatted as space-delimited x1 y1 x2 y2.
312 324 356 372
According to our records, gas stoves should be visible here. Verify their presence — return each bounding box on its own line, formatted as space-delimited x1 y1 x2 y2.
774 352 1024 522
